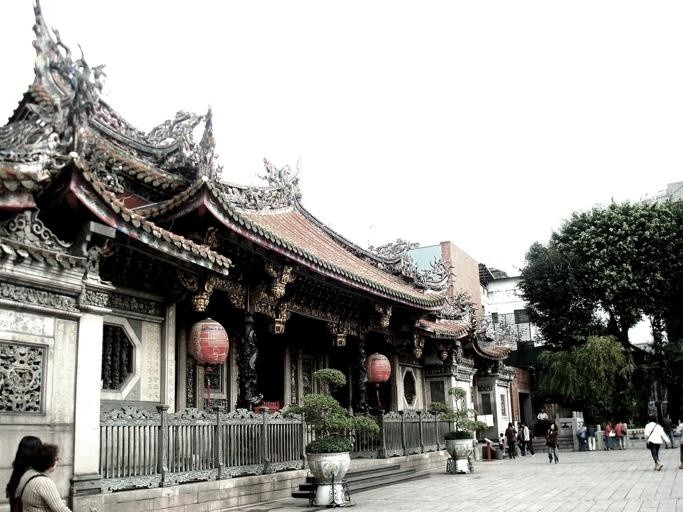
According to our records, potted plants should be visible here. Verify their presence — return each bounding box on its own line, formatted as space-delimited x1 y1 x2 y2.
427 387 489 474
282 367 382 509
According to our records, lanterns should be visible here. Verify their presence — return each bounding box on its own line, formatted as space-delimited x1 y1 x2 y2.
187 317 229 408
364 351 392 408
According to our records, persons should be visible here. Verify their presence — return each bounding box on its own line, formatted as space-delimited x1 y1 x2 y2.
576 425 586 451
535 406 548 434
521 422 535 456
585 423 595 450
504 422 517 458
542 422 560 464
642 415 673 471
12 443 74 512
604 420 615 451
613 420 626 450
515 422 524 455
662 413 677 449
675 415 683 469
498 432 505 451
4 434 43 511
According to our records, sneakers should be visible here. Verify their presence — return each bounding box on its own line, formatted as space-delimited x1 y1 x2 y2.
653 464 663 470
